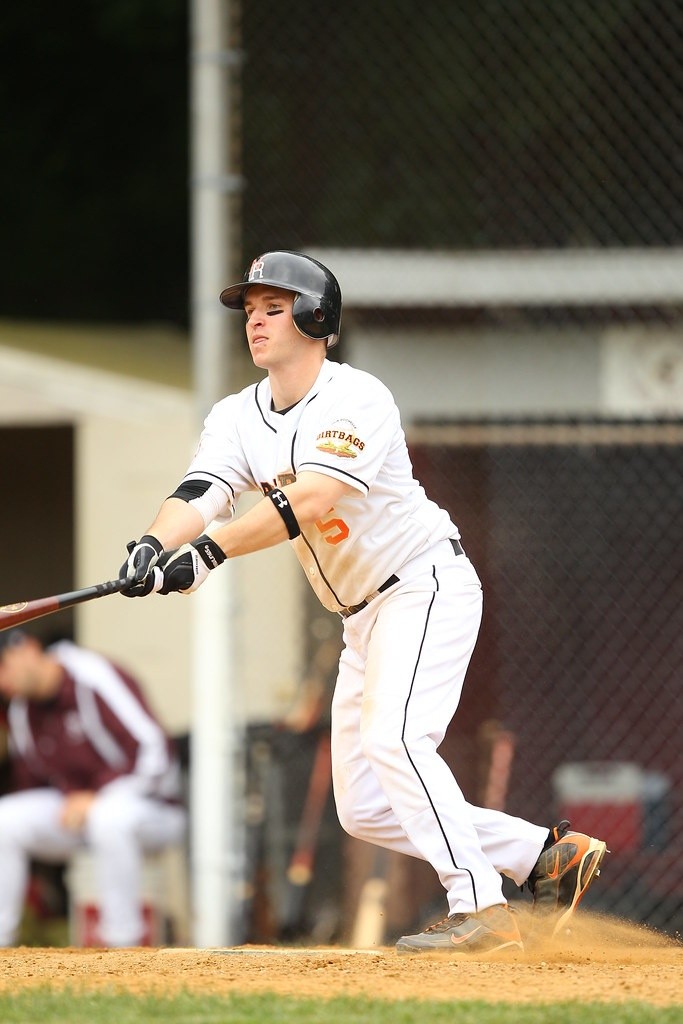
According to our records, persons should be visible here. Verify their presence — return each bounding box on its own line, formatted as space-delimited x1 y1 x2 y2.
121 251 605 963
0 627 182 948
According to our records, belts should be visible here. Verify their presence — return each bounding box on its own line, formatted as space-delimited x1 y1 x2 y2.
339 539 463 619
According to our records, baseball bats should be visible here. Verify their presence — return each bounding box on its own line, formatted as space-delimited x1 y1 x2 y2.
0 577 138 632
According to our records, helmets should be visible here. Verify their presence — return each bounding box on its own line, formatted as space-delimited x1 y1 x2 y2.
220 249 342 349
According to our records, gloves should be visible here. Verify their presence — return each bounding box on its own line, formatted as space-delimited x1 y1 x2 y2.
119 535 166 597
155 534 227 594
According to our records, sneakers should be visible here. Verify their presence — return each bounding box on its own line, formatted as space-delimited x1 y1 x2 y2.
396 905 526 964
521 820 611 950
